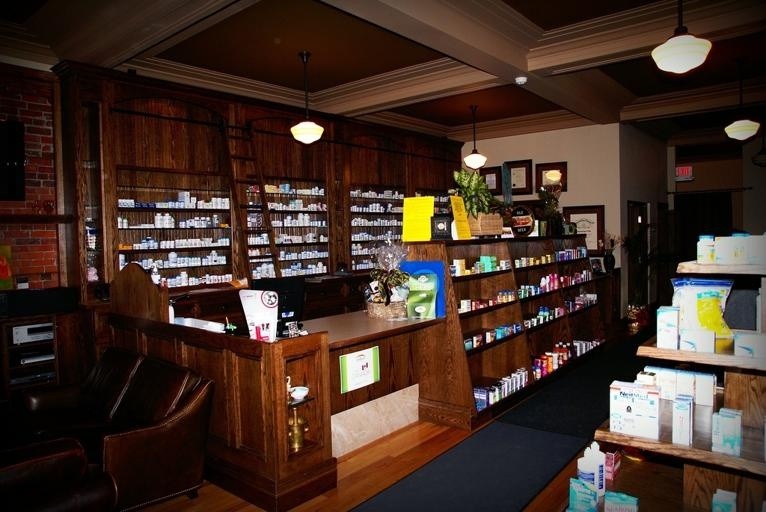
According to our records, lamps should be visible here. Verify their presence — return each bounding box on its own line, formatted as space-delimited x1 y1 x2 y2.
650 0 713 75
463 104 487 170
723 54 760 142
289 50 325 145
750 135 766 167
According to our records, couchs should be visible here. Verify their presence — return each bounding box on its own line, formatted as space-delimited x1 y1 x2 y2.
10 343 218 512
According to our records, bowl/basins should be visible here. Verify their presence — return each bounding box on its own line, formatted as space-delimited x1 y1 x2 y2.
290 386 310 400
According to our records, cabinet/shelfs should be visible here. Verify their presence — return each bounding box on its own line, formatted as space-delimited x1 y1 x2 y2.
2 320 60 400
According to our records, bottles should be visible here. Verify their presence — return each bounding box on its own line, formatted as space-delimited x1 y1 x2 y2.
291 406 299 425
587 441 608 499
116 189 233 287
575 447 601 499
245 182 331 279
698 235 716 265
349 187 405 270
497 272 573 401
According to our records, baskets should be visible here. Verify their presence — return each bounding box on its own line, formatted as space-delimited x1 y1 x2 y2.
367 277 407 319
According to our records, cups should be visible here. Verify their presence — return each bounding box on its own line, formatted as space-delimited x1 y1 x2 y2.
287 415 307 451
169 305 174 324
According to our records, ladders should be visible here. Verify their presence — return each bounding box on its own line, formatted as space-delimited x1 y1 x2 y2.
220 118 281 287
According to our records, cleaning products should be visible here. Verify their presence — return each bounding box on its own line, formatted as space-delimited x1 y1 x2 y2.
577 439 605 497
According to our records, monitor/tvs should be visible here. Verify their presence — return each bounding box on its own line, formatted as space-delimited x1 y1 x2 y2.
250 276 306 337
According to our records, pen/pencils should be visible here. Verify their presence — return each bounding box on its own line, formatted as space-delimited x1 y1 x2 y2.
226 317 229 325
